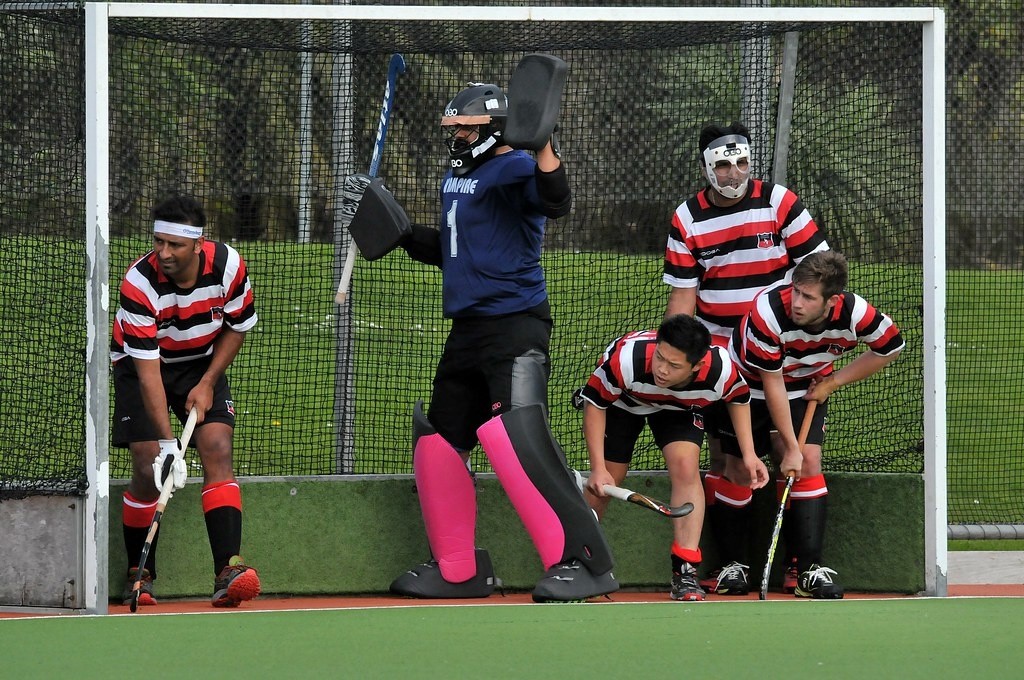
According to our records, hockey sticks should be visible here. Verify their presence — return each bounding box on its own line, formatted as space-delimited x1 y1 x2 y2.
334 53 406 305
130 406 198 613
581 476 694 517
759 399 817 600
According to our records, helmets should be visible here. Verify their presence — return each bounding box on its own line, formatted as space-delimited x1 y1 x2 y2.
699 121 752 198
441 81 508 177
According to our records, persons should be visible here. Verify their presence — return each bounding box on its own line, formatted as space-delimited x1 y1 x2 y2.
715 249 906 600
661 123 830 594
344 54 619 601
110 197 261 608
583 314 769 602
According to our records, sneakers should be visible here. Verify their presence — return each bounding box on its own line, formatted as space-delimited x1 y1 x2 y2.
120 566 158 606
794 563 845 600
715 561 750 595
211 565 263 607
670 554 706 600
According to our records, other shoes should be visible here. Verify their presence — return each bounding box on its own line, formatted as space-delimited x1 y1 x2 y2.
389 549 496 597
533 560 619 598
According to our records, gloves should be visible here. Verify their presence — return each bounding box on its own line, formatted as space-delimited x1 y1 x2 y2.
152 439 188 498
